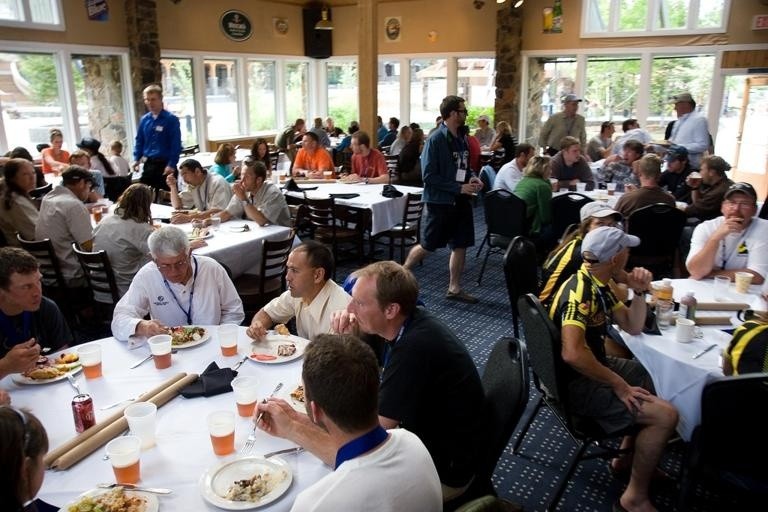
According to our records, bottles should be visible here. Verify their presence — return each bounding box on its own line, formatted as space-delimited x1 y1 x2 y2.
656 278 673 315
550 176 560 192
277 153 286 180
678 290 697 321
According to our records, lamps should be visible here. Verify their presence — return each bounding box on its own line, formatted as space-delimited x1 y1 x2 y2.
315 0 336 31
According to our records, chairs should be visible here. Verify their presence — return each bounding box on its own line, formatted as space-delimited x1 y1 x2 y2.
501 236 541 336
546 191 593 247
511 293 635 512
441 333 529 508
476 187 527 284
673 374 768 510
626 201 687 272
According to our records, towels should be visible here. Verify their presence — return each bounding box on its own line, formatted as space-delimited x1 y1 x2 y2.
181 361 239 398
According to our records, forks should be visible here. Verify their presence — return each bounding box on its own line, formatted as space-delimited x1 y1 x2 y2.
242 398 268 454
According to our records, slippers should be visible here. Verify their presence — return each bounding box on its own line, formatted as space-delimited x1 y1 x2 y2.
612 498 627 512
607 463 670 482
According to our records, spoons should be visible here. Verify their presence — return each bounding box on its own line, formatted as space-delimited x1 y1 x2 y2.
133 354 152 370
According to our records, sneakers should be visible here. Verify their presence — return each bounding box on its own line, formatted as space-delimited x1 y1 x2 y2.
446 290 480 303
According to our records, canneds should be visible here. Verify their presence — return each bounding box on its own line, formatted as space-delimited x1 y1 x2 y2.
71 393 96 433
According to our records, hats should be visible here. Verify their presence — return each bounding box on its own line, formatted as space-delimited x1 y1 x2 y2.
476 115 490 124
75 138 100 148
566 93 757 263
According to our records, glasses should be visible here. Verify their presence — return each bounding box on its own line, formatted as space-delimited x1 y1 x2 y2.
455 109 467 114
72 174 93 179
154 256 189 271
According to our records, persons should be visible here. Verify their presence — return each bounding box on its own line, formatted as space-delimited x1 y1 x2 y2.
0 245 71 379
722 318 767 378
86 183 244 341
249 331 445 511
0 404 62 511
327 257 486 490
684 182 766 284
247 242 353 340
129 85 180 201
468 93 734 250
1 128 129 291
166 138 292 228
273 116 425 238
540 200 621 310
404 95 484 303
547 226 680 512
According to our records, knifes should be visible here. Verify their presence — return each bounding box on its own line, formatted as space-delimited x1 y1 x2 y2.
63 372 80 395
98 483 171 494
234 358 246 372
264 447 303 459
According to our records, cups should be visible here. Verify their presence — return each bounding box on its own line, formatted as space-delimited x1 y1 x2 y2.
211 216 220 232
734 271 754 294
471 184 481 196
689 178 702 187
607 183 617 195
76 323 259 485
576 183 586 193
712 276 731 302
675 319 696 343
272 167 348 187
91 198 109 223
657 299 674 331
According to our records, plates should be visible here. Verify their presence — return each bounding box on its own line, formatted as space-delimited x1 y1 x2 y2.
11 358 82 385
144 326 209 349
202 453 294 511
58 486 159 512
183 229 214 240
244 335 306 364
